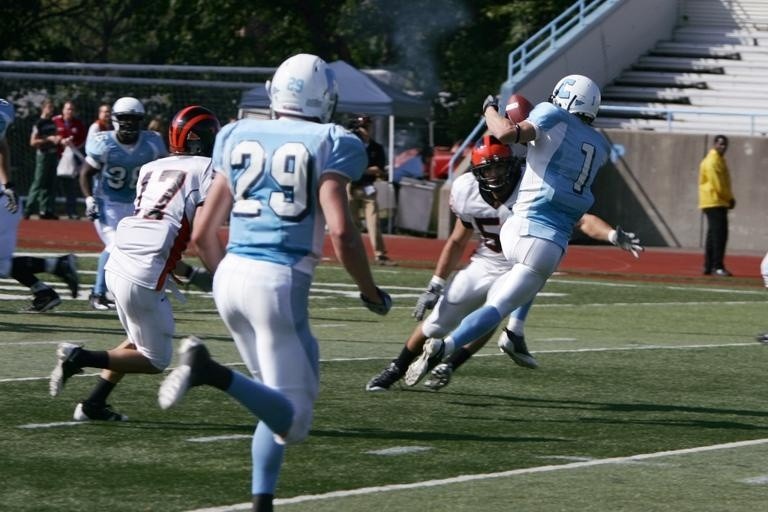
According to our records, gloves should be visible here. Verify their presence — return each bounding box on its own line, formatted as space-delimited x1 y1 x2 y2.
85 197 101 221
360 287 392 316
189 266 214 293
411 281 442 322
4 186 19 215
611 225 645 259
482 95 499 116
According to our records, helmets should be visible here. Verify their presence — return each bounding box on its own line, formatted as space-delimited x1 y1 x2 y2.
110 96 145 140
470 135 521 193
264 52 339 123
168 104 221 155
548 74 601 123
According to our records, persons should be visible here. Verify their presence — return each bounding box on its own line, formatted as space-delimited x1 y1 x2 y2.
365 135 644 393
404 74 611 390
755 253 768 343
48 104 222 422
156 54 392 512
698 135 736 276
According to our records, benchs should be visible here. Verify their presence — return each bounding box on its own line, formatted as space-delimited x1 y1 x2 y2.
588 23 768 134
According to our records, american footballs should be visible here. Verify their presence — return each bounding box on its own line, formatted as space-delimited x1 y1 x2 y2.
505 93 534 124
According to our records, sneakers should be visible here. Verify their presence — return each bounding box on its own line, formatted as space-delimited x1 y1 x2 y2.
157 335 209 410
73 401 130 423
374 254 401 267
423 361 452 390
366 364 406 391
48 342 84 397
56 254 79 298
21 287 61 313
702 267 733 277
404 337 445 388
22 208 82 220
497 326 539 369
89 290 116 310
